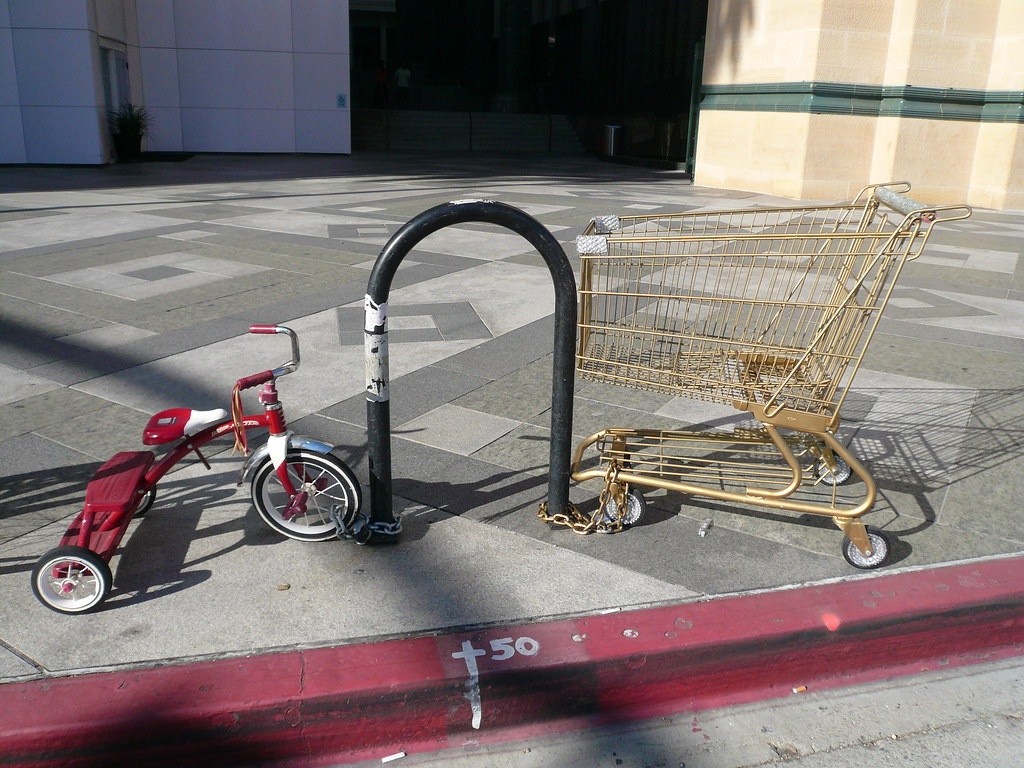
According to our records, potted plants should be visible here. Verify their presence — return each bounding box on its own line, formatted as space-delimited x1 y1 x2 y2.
105 103 159 165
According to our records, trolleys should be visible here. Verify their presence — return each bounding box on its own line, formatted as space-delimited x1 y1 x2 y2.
569 182 973 563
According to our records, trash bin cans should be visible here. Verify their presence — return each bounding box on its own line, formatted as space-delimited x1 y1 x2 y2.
598 123 624 161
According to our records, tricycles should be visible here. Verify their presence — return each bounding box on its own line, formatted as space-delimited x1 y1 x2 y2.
28 325 361 613
